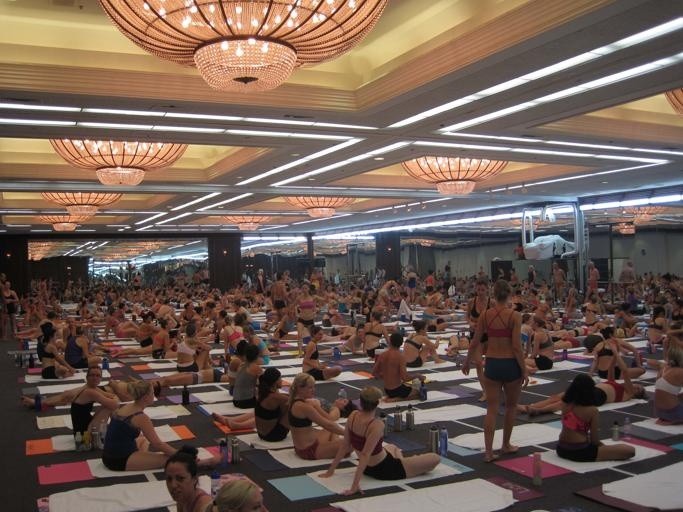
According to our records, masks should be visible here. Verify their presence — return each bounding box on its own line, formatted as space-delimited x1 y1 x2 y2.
277 381 283 389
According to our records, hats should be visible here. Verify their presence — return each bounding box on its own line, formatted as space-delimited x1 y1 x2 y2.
138 310 151 317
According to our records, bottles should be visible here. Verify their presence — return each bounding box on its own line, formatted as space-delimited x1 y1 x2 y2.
459 332 469 349
563 350 567 360
102 357 109 370
393 405 402 432
428 424 438 453
91 426 99 449
29 353 34 368
623 418 631 440
83 431 90 450
379 410 387 438
75 432 83 450
182 384 190 405
456 331 460 339
231 437 239 462
34 387 42 411
533 452 542 485
334 347 340 361
420 381 427 400
220 440 228 473
406 404 414 430
439 425 448 456
613 421 619 441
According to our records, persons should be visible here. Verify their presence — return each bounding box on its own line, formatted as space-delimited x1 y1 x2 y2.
477 266 487 277
287 373 354 461
3 280 19 339
403 320 445 368
212 397 356 431
0 272 9 341
556 373 635 462
525 316 554 370
509 261 653 326
303 324 343 380
514 337 653 417
255 366 292 442
204 479 268 512
372 332 426 403
176 324 210 371
609 259 682 341
243 324 271 366
318 264 462 318
102 376 226 472
317 322 366 356
110 331 179 358
127 368 236 388
288 282 318 358
588 328 647 379
497 265 505 280
655 340 683 426
462 281 529 462
69 365 120 441
465 278 493 403
445 334 471 359
164 445 212 512
65 325 103 369
548 337 588 349
22 269 286 336
363 311 391 361
318 386 442 495
233 344 263 408
38 328 75 379
565 327 587 337
18 378 162 408
228 339 248 396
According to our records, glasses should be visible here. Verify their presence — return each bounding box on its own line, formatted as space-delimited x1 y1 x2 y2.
88 373 102 378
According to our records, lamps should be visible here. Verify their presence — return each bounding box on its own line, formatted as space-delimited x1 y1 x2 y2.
283 197 356 219
225 216 273 230
48 140 188 186
96 0 390 93
40 192 122 216
403 157 507 196
36 216 92 232
